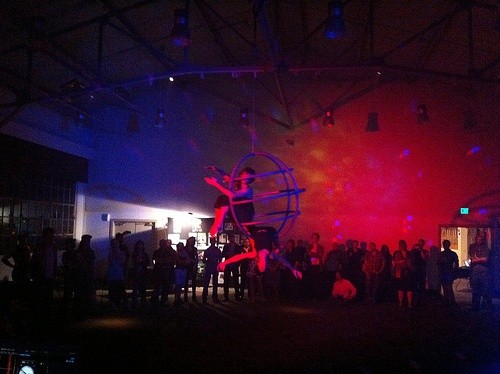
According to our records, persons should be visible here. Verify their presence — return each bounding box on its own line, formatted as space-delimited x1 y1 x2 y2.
0 226 494 336
204 165 258 235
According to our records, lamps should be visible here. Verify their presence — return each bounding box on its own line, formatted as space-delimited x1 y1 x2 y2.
322 0 347 41
238 108 250 129
172 8 193 49
420 104 429 121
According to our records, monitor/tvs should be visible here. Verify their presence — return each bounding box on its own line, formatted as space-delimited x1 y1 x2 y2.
0 346 77 373
